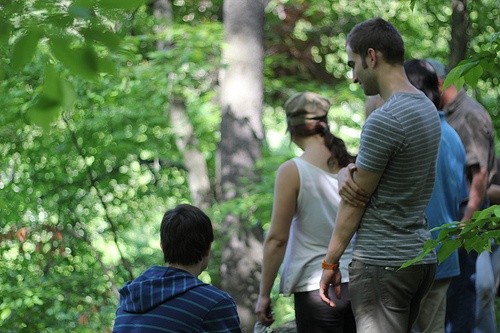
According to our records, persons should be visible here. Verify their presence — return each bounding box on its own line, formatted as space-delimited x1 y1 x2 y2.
320 19 441 333
255 92 356 333
431 60 500 333
404 59 467 333
113 204 242 333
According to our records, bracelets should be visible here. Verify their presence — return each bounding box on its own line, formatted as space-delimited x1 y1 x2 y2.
322 259 339 270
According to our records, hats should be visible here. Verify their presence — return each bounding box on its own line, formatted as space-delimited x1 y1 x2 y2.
427 60 446 79
285 92 331 134
